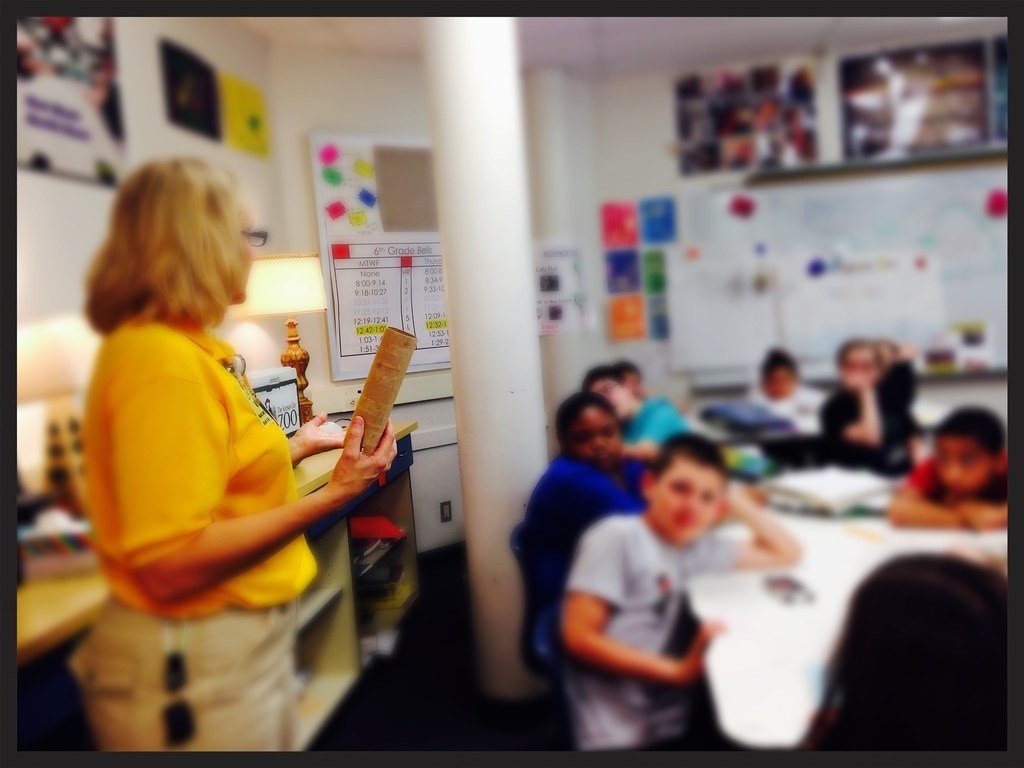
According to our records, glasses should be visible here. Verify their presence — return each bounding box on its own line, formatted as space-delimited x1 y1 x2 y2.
241 230 269 247
162 649 195 748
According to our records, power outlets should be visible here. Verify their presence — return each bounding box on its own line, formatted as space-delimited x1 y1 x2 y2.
439 500 452 523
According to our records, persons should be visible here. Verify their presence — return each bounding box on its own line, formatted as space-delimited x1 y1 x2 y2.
67 157 395 748
514 334 1006 751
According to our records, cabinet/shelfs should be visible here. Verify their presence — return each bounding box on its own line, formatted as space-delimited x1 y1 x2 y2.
17 422 421 752
